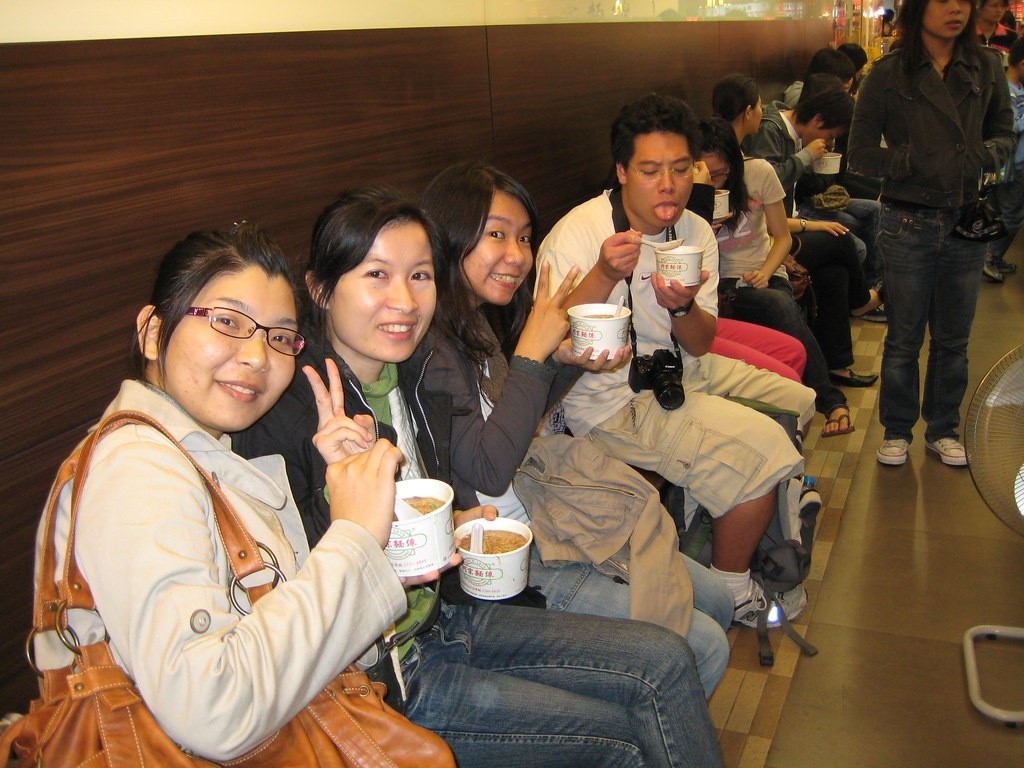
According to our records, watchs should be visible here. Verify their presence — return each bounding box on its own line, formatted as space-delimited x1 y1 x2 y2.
667 297 694 318
800 219 807 232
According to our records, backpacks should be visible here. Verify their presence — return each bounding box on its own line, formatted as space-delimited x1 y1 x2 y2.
661 396 824 597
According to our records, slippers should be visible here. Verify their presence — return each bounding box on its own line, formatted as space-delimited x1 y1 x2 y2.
820 406 856 437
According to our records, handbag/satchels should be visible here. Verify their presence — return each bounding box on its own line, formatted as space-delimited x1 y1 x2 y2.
950 193 1009 242
0 410 458 768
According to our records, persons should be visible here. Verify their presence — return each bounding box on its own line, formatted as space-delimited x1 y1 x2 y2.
0 221 464 768
398 161 736 705
533 44 887 629
882 0 1024 283
228 188 729 768
846 0 1018 467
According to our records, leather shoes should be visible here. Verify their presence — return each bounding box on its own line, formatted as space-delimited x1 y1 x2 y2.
830 369 878 388
850 281 887 319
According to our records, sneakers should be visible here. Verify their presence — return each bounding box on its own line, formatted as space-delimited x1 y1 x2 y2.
982 259 1016 282
876 437 970 466
730 578 809 629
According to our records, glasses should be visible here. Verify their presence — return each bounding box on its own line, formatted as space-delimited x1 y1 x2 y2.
625 159 698 181
181 307 306 358
712 160 731 182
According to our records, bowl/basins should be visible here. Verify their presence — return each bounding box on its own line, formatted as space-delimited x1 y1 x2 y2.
568 304 630 360
713 190 730 219
656 247 704 286
455 519 532 598
383 477 456 577
813 153 842 174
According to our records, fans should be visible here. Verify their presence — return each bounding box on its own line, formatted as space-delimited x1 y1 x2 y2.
962 342 1024 731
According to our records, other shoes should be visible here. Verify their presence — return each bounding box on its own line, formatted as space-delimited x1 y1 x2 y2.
862 308 888 322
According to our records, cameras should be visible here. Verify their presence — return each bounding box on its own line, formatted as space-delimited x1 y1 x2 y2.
628 348 685 410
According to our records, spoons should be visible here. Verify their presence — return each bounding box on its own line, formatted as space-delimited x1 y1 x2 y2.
640 239 684 250
394 496 422 521
